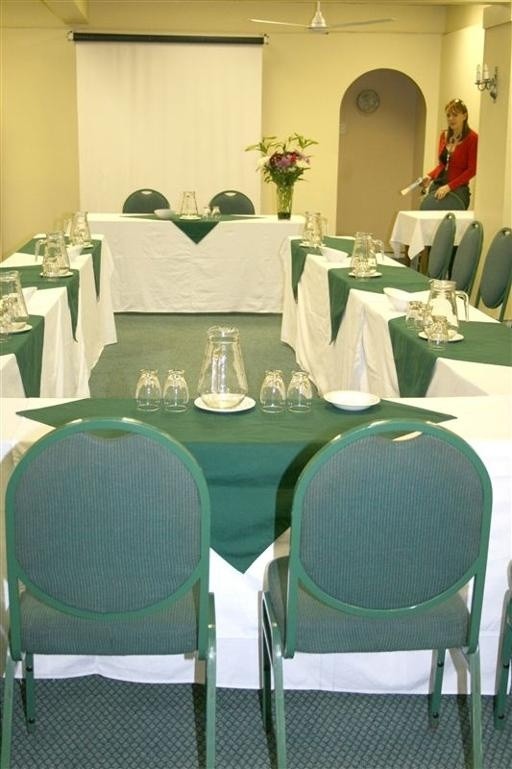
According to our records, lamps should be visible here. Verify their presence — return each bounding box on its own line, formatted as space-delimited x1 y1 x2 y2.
476 64 499 99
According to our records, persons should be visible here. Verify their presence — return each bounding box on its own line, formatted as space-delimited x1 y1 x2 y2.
415 100 477 280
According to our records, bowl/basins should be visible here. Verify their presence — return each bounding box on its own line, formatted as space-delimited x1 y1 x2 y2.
153 209 175 219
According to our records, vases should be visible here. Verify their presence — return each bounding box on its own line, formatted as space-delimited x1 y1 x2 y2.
276 185 294 220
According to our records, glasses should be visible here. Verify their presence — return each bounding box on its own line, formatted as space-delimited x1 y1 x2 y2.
449 99 465 110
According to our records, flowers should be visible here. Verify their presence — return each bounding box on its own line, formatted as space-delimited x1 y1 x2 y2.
245 133 317 211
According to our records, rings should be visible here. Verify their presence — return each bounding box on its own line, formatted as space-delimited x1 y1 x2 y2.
441 192 444 194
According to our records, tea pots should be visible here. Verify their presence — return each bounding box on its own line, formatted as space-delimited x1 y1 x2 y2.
0 208 91 323
181 191 198 218
303 211 470 336
198 325 248 409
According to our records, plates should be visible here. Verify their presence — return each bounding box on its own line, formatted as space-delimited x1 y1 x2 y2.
324 389 385 410
194 394 257 413
298 242 324 248
418 330 465 342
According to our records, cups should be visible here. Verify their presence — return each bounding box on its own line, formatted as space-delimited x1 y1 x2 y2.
137 368 187 415
256 370 314 414
203 206 221 220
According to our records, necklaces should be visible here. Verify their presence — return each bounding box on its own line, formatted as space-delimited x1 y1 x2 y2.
445 134 455 171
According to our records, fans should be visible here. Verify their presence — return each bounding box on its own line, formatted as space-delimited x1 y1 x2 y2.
247 1 393 35
